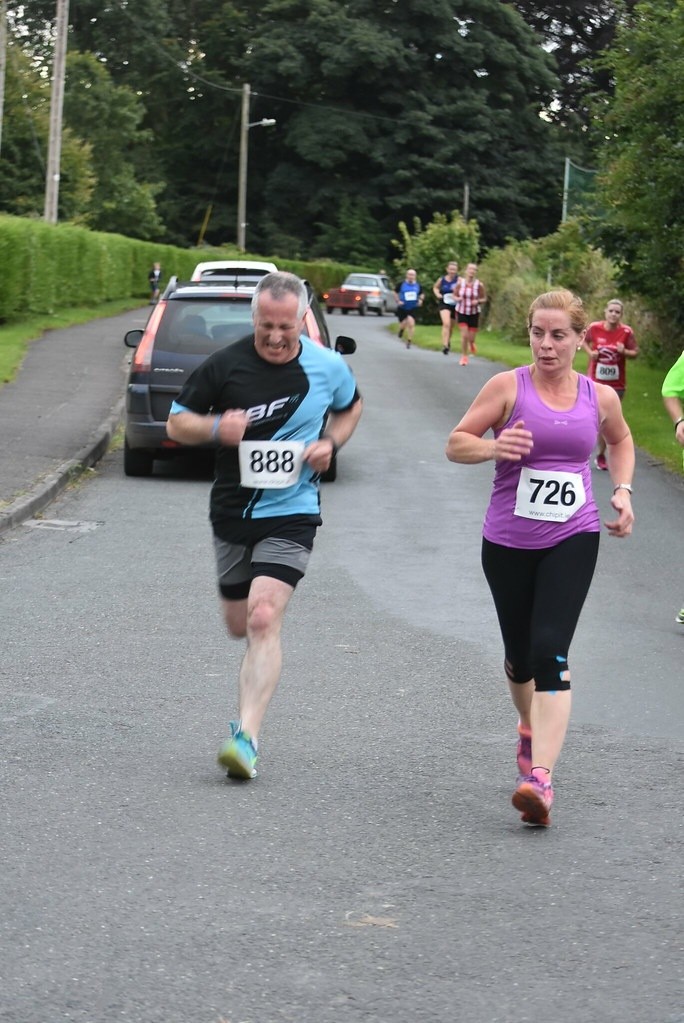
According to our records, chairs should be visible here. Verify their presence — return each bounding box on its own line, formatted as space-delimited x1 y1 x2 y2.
168 314 214 354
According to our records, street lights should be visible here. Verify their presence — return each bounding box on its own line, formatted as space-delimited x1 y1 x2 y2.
238 119 276 254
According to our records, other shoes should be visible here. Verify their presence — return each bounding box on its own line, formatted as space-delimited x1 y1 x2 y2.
469 344 476 354
442 347 449 354
398 330 403 337
459 356 467 366
407 341 410 347
676 609 684 623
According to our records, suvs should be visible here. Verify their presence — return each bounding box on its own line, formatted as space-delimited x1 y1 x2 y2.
340 272 397 315
124 277 357 477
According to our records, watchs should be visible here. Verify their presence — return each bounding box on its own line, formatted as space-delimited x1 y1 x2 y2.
613 484 633 495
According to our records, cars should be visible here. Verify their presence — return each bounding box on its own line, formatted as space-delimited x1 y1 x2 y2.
192 261 278 283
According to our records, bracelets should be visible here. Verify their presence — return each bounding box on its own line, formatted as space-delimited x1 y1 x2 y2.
477 299 481 304
322 434 338 457
210 413 221 443
674 419 684 430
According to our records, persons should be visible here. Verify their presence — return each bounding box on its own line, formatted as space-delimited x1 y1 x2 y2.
447 290 636 827
662 350 684 622
432 262 463 354
166 270 362 780
392 270 425 348
149 262 162 305
580 300 639 471
452 264 488 366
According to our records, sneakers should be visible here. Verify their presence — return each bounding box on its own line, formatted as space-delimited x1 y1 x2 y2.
217 719 258 780
516 718 534 778
511 766 554 827
595 454 607 470
